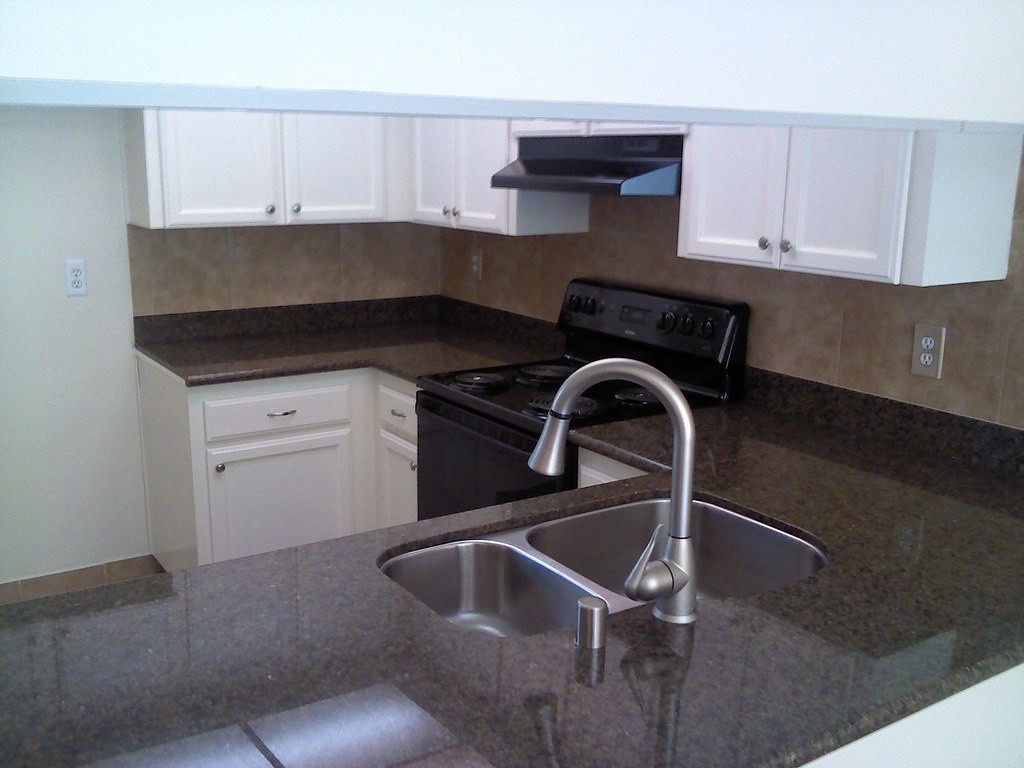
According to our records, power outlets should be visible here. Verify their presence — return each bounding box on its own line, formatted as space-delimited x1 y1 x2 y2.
911 323 946 379
66 259 85 298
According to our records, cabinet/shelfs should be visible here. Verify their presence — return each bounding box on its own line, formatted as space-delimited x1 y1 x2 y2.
378 385 418 528
204 384 354 563
409 116 594 235
676 124 1021 288
122 106 388 227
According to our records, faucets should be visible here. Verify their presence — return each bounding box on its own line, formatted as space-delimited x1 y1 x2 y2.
528 358 699 623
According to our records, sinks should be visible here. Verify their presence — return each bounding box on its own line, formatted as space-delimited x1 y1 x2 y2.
376 538 616 638
525 489 832 604
